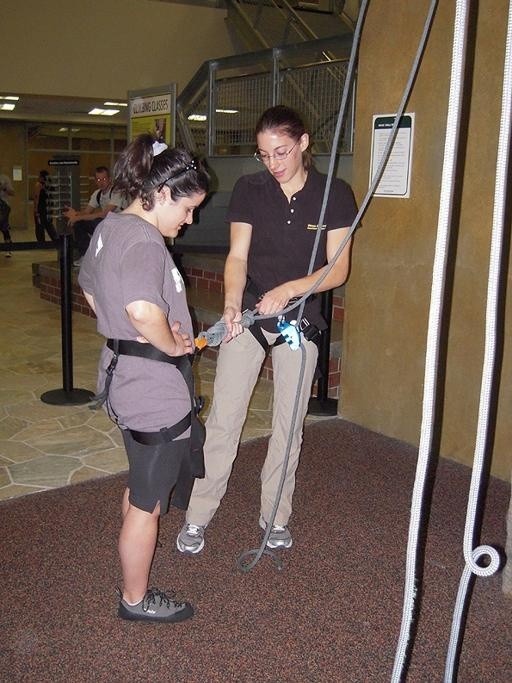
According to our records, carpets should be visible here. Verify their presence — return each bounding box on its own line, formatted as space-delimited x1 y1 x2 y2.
0 418 512 683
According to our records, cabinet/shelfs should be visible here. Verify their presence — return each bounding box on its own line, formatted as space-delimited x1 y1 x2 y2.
46 176 88 218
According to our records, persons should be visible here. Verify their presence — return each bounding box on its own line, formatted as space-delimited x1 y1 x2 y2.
62 166 128 266
0 173 17 257
33 170 57 243
77 128 211 624
173 103 362 551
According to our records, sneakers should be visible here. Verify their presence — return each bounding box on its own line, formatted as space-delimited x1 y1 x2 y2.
115 588 192 622
257 511 297 552
73 253 85 268
174 512 209 558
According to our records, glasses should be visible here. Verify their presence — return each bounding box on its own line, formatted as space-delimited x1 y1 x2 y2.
156 154 201 194
252 136 308 163
